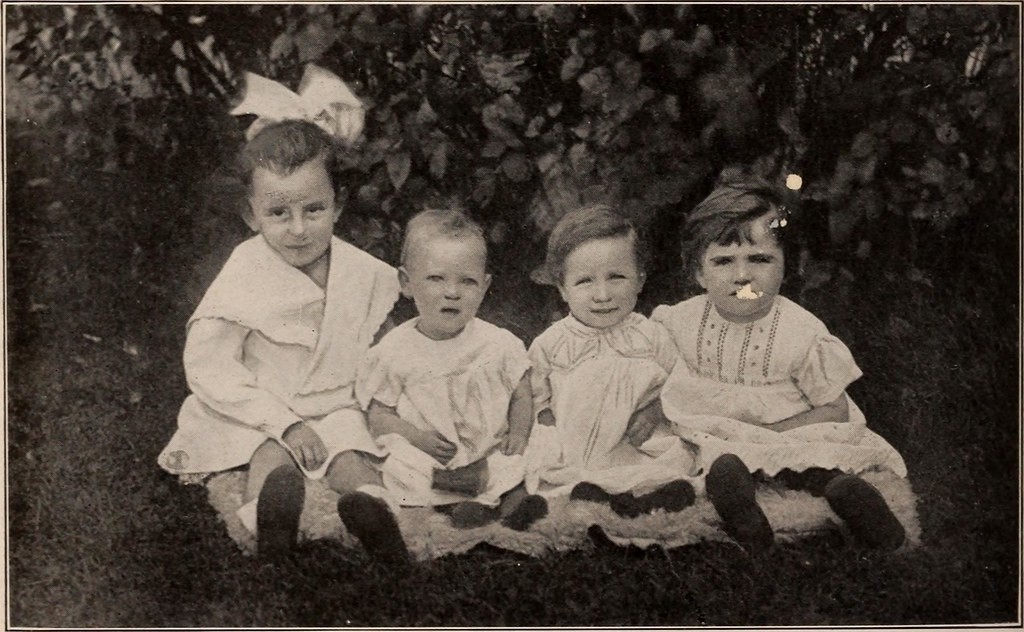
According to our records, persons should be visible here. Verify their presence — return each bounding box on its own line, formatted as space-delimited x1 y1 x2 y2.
355 209 565 531
648 179 908 561
158 74 431 580
529 206 704 519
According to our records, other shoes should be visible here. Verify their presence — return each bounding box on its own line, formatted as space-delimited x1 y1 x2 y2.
255 466 306 559
571 480 696 517
450 501 498 532
504 496 549 533
706 455 776 546
337 492 428 595
822 474 898 545
